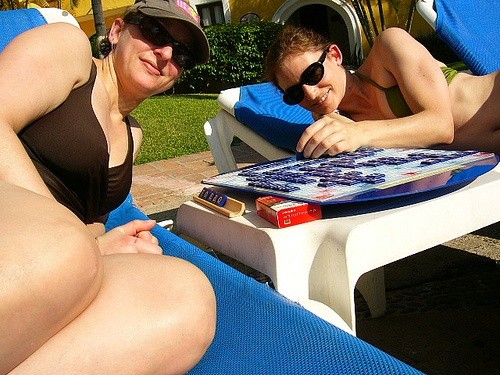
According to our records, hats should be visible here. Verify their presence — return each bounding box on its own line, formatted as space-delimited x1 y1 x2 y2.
271 0 363 69
121 0 210 65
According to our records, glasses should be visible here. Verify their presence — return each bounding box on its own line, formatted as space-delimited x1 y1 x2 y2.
125 16 197 70
283 50 327 106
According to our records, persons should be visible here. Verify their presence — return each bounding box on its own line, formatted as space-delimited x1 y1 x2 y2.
266 26 500 159
0 0 217 375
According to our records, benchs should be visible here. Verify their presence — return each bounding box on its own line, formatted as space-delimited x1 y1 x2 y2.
203 69 357 180
0 8 427 375
415 0 500 75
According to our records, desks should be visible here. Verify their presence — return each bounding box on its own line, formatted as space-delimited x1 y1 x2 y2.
171 146 500 338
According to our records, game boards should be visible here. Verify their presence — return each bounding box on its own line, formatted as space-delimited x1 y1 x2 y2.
201 144 496 204
